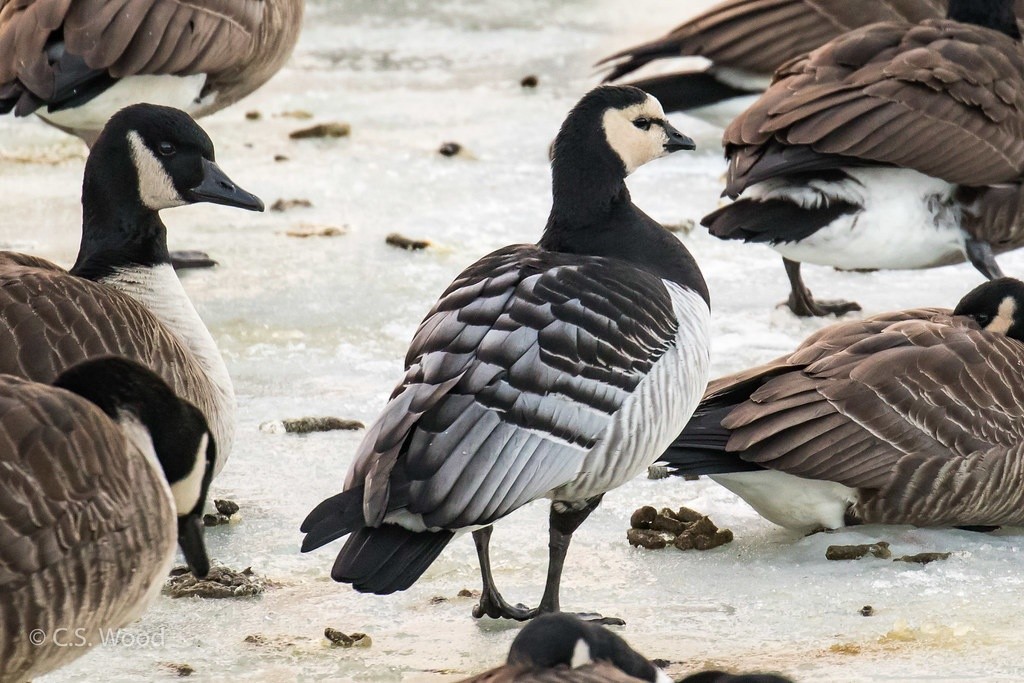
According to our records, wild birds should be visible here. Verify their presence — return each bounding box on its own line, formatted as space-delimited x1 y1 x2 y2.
300 84 719 627
461 613 794 683
698 0 1024 317
2 103 265 597
3 353 218 681
592 0 947 119
1 1 303 268
646 276 1024 535
